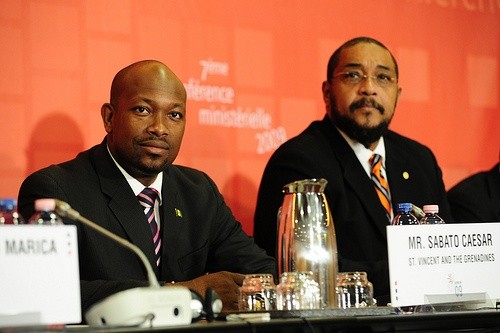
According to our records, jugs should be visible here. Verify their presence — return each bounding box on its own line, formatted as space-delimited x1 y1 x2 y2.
276 177 339 309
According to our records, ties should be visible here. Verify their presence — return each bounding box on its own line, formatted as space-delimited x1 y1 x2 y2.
135 186 160 273
367 152 393 225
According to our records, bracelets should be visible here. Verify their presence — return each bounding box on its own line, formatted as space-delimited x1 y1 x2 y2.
171 281 175 286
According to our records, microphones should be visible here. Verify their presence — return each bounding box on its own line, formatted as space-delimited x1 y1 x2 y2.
52 199 192 327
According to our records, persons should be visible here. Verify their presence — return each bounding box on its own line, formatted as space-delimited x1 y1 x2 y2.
16 59 279 326
252 36 456 313
447 160 500 223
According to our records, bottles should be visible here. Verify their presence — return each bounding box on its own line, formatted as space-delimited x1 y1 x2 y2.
0 198 25 224
420 205 445 224
29 198 64 225
392 203 420 225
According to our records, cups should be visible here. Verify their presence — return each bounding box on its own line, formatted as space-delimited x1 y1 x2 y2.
276 271 321 310
335 271 373 308
238 273 277 312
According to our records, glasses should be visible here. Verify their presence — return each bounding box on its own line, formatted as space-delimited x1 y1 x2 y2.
330 69 397 88
